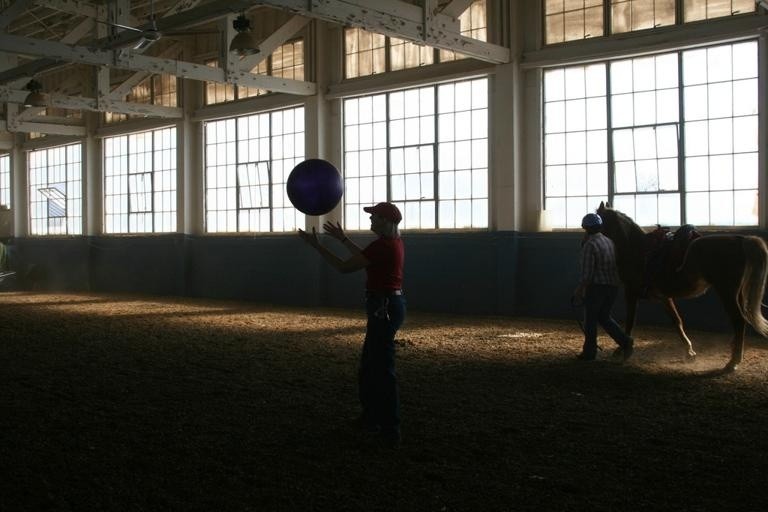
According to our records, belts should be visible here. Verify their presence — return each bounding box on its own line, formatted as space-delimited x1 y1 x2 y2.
365 289 401 299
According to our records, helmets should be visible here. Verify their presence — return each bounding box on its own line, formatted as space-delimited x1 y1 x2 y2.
582 214 603 227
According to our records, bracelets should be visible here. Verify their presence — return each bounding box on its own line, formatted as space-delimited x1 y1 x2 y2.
341 236 348 243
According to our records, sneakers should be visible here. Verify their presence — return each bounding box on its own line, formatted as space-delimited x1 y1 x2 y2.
575 353 595 360
623 335 633 361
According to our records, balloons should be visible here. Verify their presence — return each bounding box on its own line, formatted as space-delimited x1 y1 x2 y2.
286 159 345 216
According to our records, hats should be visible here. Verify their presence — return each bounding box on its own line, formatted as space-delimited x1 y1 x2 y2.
363 202 402 224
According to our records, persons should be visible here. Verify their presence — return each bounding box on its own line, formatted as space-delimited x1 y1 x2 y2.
575 213 634 361
298 202 406 448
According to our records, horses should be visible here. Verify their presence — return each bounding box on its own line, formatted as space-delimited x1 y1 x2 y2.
578 202 767 371
